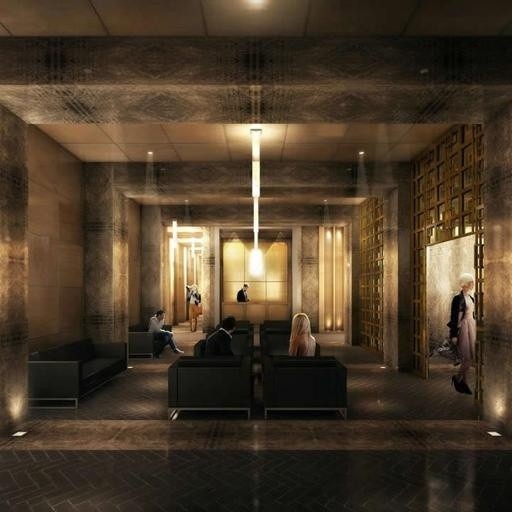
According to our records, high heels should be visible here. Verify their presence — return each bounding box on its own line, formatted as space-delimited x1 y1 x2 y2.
451 375 471 396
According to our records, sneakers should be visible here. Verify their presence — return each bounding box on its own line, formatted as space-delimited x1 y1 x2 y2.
174 348 186 355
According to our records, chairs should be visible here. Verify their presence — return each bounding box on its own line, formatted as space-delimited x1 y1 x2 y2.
168 320 347 420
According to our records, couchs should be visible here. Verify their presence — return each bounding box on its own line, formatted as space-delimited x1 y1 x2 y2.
29 337 127 410
129 325 172 360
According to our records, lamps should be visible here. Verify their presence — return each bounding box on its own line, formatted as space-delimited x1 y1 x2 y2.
251 129 261 249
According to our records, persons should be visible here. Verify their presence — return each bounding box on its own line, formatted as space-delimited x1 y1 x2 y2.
288 312 317 356
186 284 203 333
448 273 478 396
205 315 237 357
237 283 250 302
144 309 185 360
